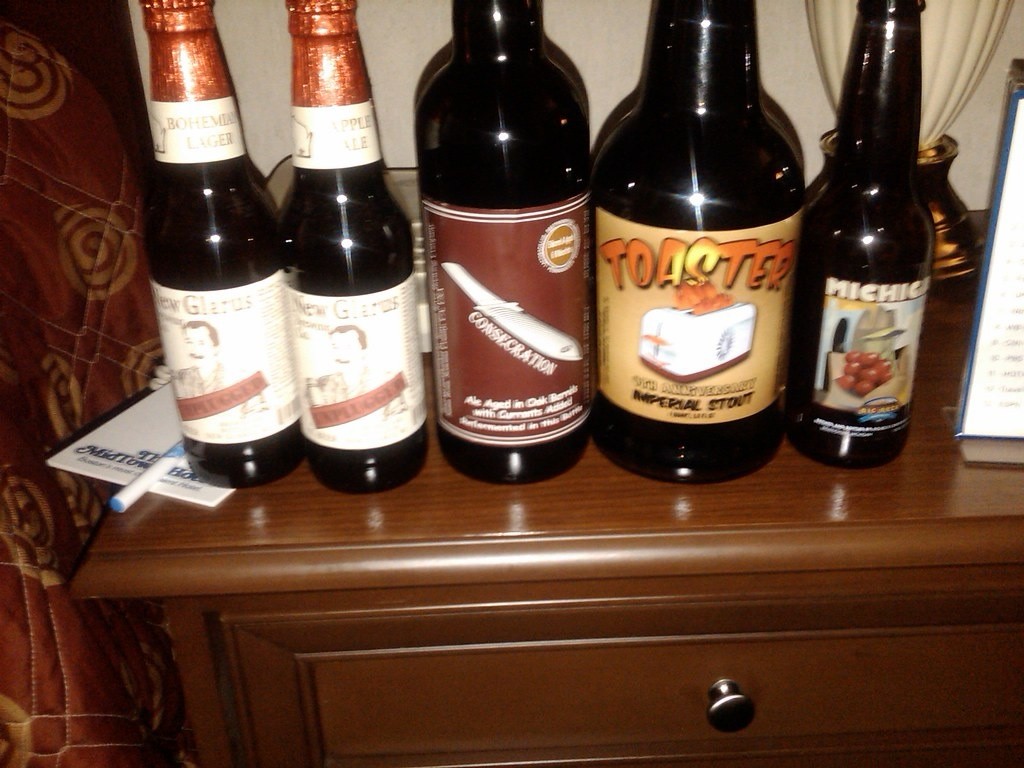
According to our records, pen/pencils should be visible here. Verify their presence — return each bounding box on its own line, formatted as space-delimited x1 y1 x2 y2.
106 439 188 514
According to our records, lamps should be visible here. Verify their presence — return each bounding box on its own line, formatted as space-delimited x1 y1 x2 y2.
804 0 1015 290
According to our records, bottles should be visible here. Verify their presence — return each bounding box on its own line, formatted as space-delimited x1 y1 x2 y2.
282 1 429 495
136 0 306 488
589 0 807 484
414 0 595 485
784 0 936 469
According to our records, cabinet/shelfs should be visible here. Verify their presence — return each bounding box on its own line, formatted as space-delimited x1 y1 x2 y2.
67 209 1024 768
